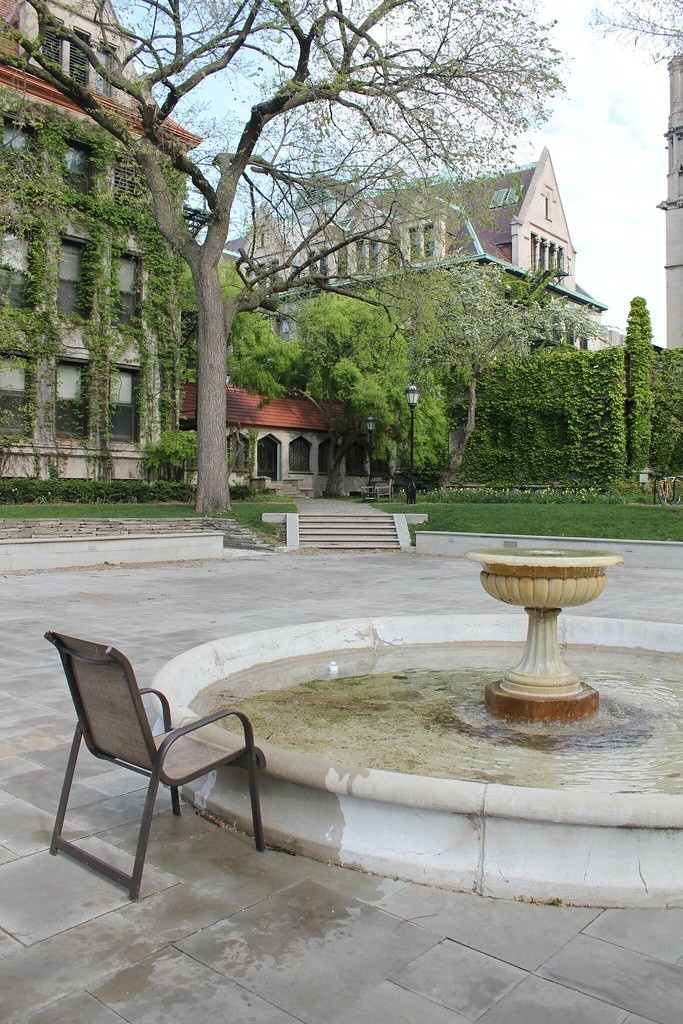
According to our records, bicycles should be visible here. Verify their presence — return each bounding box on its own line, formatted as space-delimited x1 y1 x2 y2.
658 475 675 505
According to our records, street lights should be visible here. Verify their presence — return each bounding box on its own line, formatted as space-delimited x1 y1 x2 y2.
365 415 376 496
404 385 420 503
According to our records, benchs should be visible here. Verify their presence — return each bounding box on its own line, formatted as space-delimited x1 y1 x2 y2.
359 479 392 501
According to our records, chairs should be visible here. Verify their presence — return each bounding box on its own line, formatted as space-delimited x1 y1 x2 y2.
44 629 266 901
